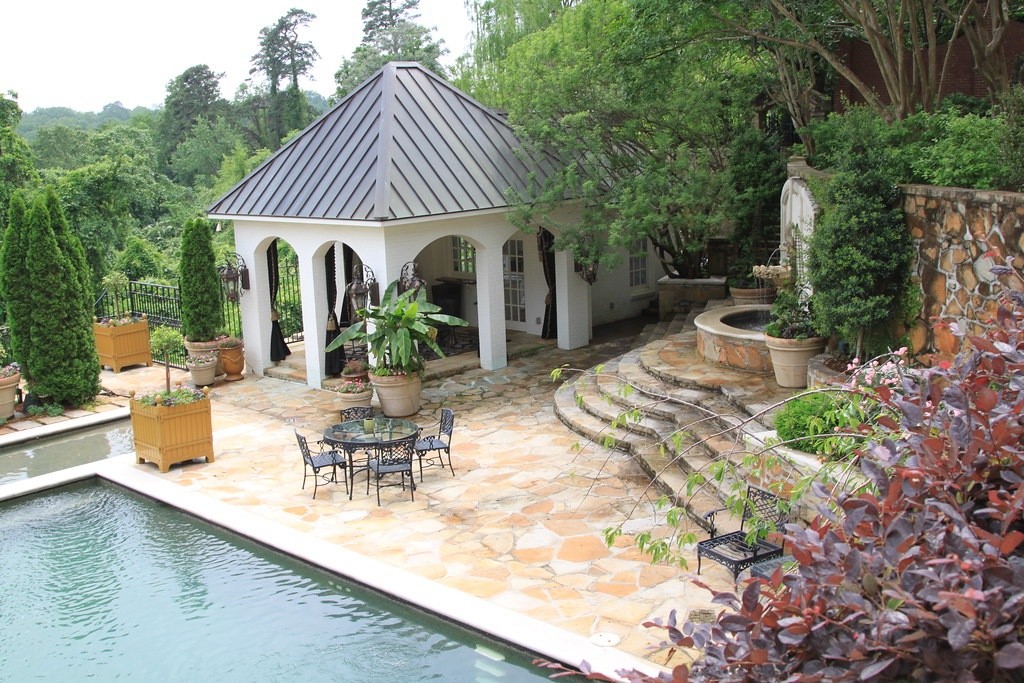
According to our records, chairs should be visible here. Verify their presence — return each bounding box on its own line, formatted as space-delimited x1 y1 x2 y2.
294 406 467 507
696 484 791 595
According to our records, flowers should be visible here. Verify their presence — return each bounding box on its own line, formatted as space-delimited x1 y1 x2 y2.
0 344 20 378
336 379 367 393
218 335 242 348
186 349 219 368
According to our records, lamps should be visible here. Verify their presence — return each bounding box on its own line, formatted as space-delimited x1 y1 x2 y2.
397 260 427 305
347 264 379 321
219 252 250 306
574 234 599 286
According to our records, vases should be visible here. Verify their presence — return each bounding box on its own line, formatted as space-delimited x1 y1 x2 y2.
337 392 373 411
185 353 218 386
217 348 245 382
0 370 21 422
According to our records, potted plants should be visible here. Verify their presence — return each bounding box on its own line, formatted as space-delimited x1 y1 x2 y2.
764 221 827 388
728 236 777 307
183 216 228 377
323 278 469 418
806 85 894 393
340 357 371 385
92 270 153 374
128 324 215 474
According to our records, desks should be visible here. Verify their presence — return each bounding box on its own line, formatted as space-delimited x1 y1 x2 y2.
324 418 418 500
